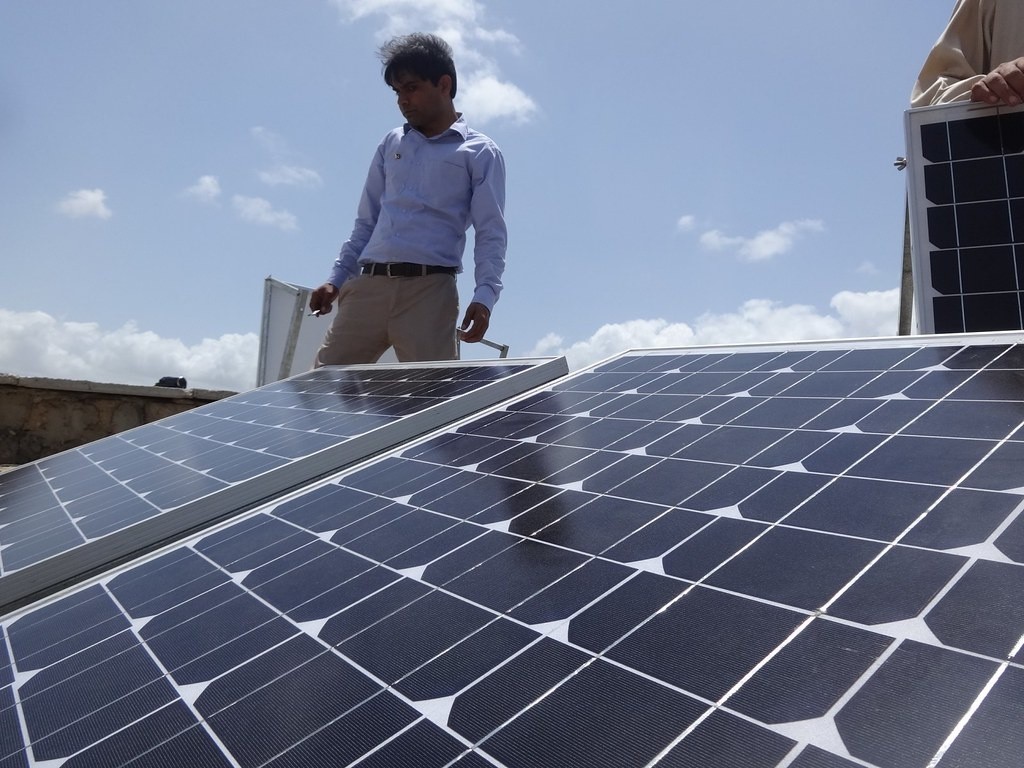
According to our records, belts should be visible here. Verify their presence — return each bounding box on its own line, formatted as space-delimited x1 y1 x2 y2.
362 262 456 277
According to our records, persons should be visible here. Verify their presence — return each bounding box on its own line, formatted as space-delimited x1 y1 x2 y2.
309 33 508 368
911 0 1023 108
